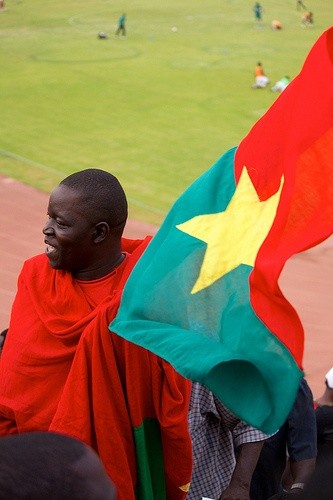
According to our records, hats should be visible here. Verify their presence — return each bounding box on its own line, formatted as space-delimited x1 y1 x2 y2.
325 367 333 389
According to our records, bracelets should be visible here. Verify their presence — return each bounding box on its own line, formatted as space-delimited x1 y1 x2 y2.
290 483 305 490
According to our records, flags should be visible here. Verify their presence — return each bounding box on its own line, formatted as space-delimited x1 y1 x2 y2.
106 27 333 434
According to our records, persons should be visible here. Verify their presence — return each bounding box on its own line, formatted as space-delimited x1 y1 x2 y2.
185 367 333 500
0 431 117 500
251 0 315 90
0 167 195 500
115 13 126 36
